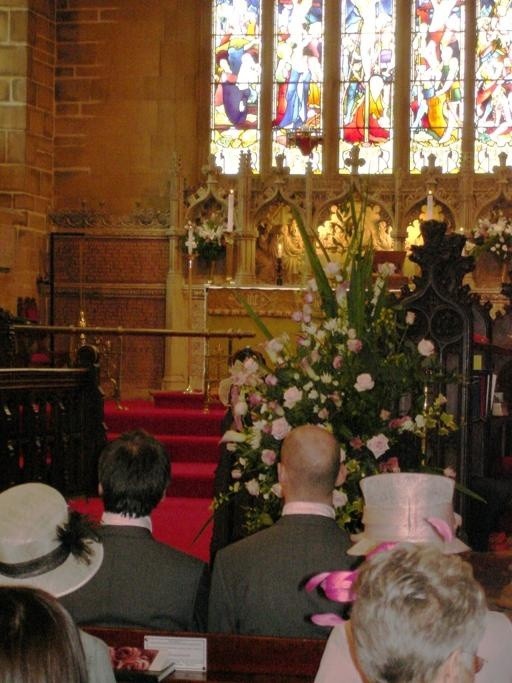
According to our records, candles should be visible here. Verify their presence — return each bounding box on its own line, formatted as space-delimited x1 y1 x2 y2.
426 190 433 221
224 189 236 286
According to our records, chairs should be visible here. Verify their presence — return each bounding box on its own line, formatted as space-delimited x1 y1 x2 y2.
76 627 328 679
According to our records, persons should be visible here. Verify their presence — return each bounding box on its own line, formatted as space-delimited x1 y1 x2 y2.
255 223 307 275
54 356 80 415
58 430 210 633
207 426 360 639
1 484 114 682
371 220 394 251
313 473 511 682
10 334 53 413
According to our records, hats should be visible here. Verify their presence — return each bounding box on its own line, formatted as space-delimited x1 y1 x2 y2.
0 482 104 599
346 472 472 556
